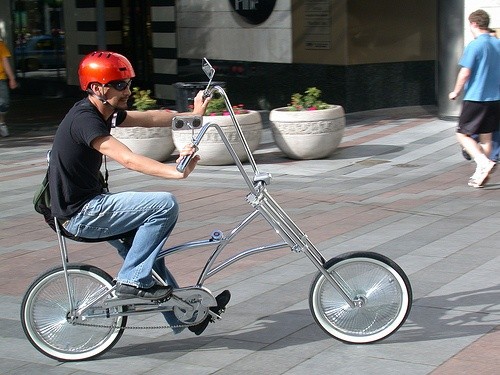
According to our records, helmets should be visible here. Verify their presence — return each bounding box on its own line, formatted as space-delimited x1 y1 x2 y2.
79 50 136 91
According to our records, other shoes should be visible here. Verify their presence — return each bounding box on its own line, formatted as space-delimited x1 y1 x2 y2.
188 290 231 336
114 282 173 300
0 123 9 136
468 157 495 187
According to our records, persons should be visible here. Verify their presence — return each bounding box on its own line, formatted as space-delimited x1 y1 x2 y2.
0 29 19 137
48 50 231 336
448 9 500 188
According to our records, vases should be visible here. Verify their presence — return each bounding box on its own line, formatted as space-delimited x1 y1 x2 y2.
269 105 346 160
172 110 262 165
110 110 178 161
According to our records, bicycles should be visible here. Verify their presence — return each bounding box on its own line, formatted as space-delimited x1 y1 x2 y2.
19 56 412 362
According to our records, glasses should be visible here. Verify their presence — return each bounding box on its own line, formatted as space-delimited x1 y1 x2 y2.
106 80 132 91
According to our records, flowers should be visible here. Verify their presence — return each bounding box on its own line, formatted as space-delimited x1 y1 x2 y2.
132 88 169 112
287 87 331 111
184 97 250 116
